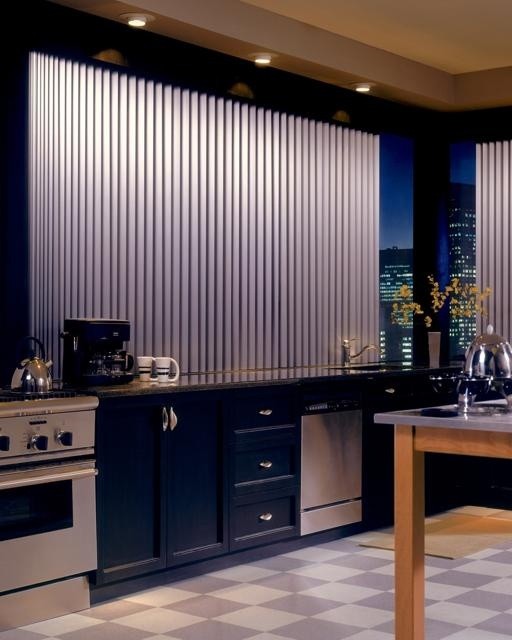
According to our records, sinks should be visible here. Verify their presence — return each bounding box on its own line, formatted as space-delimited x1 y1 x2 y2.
323 365 422 371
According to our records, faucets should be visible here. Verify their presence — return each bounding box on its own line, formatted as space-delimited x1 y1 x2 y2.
341 337 379 366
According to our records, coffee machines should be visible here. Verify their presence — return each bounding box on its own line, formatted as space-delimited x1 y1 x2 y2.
62 318 134 390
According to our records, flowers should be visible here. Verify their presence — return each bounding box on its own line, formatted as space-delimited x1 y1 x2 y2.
388 273 493 331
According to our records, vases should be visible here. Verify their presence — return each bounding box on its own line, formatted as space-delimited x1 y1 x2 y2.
428 333 441 368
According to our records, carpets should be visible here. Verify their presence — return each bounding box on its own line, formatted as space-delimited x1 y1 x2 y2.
358 513 512 560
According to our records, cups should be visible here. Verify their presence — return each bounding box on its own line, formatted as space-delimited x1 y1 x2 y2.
137 357 156 382
153 357 180 383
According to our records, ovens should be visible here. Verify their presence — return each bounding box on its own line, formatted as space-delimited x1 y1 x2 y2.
0 459 123 595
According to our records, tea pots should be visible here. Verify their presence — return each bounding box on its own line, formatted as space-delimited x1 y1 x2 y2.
10 336 54 393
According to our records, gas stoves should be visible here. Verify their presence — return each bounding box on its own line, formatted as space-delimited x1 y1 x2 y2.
0 389 99 466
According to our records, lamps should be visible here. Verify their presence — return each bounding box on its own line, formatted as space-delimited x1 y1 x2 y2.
348 82 376 95
246 51 279 66
119 11 156 28
